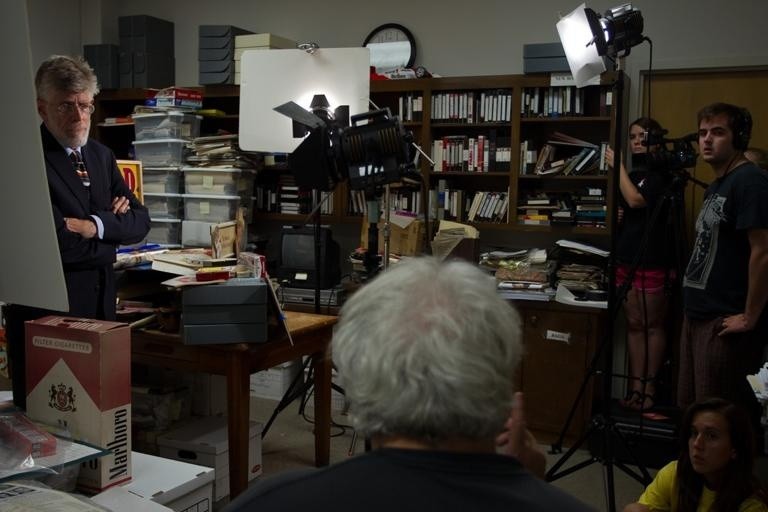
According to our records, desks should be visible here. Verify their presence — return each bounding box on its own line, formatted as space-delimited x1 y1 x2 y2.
125 311 339 508
0 386 215 512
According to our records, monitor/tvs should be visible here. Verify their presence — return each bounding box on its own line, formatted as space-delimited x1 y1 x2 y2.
276 228 326 288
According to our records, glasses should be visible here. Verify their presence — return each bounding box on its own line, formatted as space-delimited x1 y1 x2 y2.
45 101 95 115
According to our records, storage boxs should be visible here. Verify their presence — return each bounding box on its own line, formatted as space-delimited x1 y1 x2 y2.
131 113 259 247
158 413 263 502
82 13 297 89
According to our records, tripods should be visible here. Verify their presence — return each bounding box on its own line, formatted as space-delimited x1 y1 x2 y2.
260 190 346 439
551 188 690 450
543 69 653 511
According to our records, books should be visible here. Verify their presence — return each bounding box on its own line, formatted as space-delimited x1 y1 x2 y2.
104 87 267 291
280 187 386 218
384 87 609 307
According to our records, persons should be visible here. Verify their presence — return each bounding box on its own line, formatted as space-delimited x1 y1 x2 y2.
677 103 768 409
742 148 767 170
220 254 600 511
603 118 682 412
34 55 151 324
622 397 768 511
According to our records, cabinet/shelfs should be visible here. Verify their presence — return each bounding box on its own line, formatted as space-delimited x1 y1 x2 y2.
89 66 631 452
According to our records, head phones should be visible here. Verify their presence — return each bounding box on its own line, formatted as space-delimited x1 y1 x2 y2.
731 105 752 152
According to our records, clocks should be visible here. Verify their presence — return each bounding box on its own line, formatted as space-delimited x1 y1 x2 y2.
359 23 417 76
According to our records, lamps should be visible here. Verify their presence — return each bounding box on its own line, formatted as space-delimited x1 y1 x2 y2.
545 1 658 511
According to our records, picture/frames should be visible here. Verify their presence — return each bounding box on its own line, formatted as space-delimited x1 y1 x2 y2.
113 160 144 208
